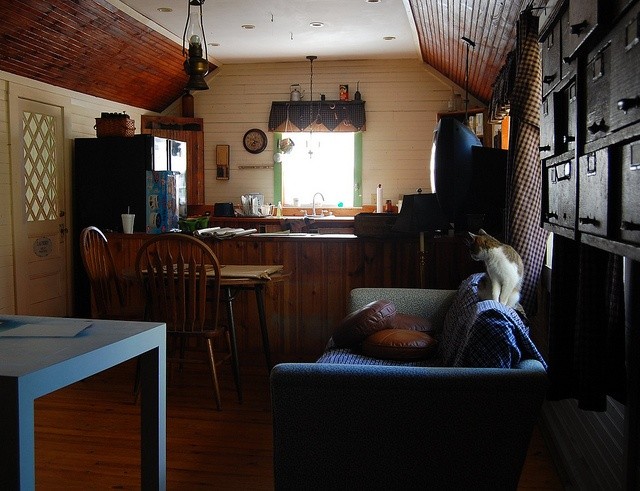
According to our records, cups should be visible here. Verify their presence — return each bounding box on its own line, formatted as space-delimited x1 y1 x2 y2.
121 213 135 235
260 205 269 215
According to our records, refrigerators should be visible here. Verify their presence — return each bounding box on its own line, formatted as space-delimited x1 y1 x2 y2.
71 136 187 317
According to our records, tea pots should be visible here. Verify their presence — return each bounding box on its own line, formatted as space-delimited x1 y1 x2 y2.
289 84 305 102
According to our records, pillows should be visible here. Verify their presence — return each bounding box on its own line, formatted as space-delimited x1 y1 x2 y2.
365 329 432 355
330 300 397 346
383 312 435 329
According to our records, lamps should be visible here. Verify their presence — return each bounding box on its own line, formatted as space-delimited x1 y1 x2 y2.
306 58 320 159
391 192 454 289
183 0 209 89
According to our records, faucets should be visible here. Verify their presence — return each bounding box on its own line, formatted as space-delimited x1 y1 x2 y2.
312 192 324 216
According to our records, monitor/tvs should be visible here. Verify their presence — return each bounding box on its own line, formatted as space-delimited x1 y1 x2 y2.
429 113 483 206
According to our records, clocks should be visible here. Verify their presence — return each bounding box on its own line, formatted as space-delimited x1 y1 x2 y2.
243 128 267 154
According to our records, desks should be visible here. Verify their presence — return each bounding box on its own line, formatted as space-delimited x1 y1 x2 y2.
142 264 292 405
0 316 168 490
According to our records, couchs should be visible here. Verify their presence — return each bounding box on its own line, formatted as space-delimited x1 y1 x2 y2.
269 287 548 488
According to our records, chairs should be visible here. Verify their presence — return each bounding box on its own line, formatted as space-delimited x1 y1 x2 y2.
134 232 235 411
79 226 123 319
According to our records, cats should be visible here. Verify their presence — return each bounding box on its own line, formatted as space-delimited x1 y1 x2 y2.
467 229 524 308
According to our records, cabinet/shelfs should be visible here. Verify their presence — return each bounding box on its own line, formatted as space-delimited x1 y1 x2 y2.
539 98 555 160
541 18 561 96
576 148 612 237
584 44 610 144
542 168 558 221
566 0 600 58
555 159 576 226
563 81 576 150
557 22 575 81
610 134 640 245
538 0 570 36
607 24 639 136
